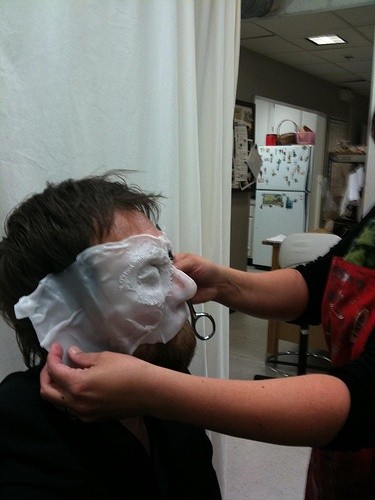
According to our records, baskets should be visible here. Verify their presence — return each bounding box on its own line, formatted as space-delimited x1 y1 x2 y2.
296 130 315 145
277 119 298 146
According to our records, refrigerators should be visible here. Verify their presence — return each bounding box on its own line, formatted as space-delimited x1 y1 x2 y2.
253 144 314 267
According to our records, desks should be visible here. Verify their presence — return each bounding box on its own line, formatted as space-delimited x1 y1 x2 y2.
261 234 336 363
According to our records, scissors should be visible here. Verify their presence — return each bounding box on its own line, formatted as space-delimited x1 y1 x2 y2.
155 223 216 340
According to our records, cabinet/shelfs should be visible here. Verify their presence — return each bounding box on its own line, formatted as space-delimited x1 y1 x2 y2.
328 151 366 235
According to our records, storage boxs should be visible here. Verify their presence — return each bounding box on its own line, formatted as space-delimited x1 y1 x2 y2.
296 133 314 143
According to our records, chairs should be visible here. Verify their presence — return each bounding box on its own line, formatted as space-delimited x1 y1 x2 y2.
253 234 341 382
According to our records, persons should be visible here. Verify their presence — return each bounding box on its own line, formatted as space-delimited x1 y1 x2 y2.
0 169 222 500
40 108 375 500
